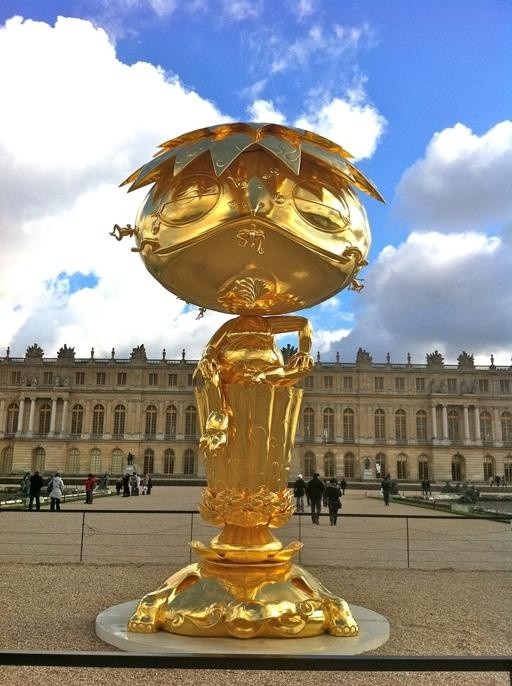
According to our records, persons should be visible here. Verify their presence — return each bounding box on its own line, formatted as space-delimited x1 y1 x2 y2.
28 471 44 510
47 473 66 511
488 475 494 486
495 475 500 486
321 478 341 526
293 474 305 512
425 480 432 496
22 472 32 508
421 480 427 495
500 475 505 486
83 473 97 504
382 477 390 507
306 473 324 524
386 473 393 492
115 472 153 497
339 479 347 494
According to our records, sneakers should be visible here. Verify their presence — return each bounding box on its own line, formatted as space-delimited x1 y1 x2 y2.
83 501 93 504
296 506 305 512
311 518 337 526
121 492 152 498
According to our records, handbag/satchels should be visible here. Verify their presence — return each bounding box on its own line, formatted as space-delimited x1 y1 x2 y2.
47 480 54 493
